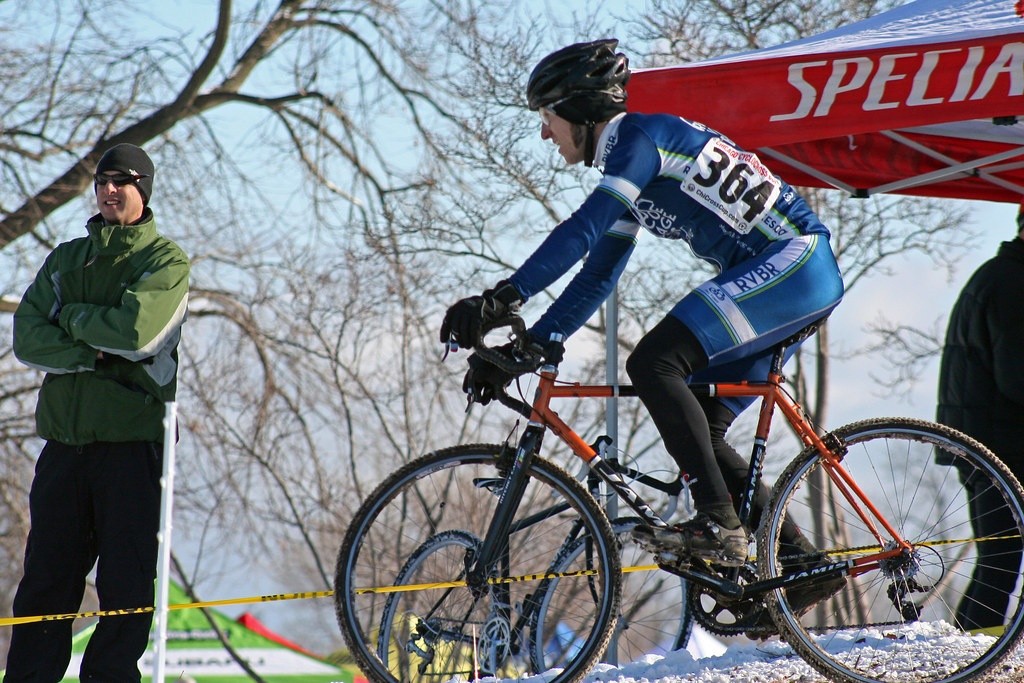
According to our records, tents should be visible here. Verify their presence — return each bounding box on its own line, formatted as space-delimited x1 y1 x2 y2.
0 575 368 683
604 0 1024 667
325 612 589 683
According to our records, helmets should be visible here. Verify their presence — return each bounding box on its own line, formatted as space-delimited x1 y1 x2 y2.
526 39 631 111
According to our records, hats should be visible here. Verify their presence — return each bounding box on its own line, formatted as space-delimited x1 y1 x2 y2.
94 143 154 205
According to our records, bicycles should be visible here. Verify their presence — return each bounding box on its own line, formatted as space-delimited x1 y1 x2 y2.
376 434 697 677
334 313 1024 683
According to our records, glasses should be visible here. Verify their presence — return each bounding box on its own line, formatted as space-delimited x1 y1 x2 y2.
539 95 575 127
92 173 150 186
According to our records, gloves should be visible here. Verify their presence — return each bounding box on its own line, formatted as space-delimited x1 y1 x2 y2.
102 351 155 364
462 333 552 406
440 280 524 350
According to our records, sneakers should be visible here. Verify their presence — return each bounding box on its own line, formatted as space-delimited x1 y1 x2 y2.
630 512 748 567
745 551 848 640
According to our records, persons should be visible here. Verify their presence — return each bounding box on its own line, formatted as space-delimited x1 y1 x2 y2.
2 142 191 683
934 198 1024 637
437 39 847 641
